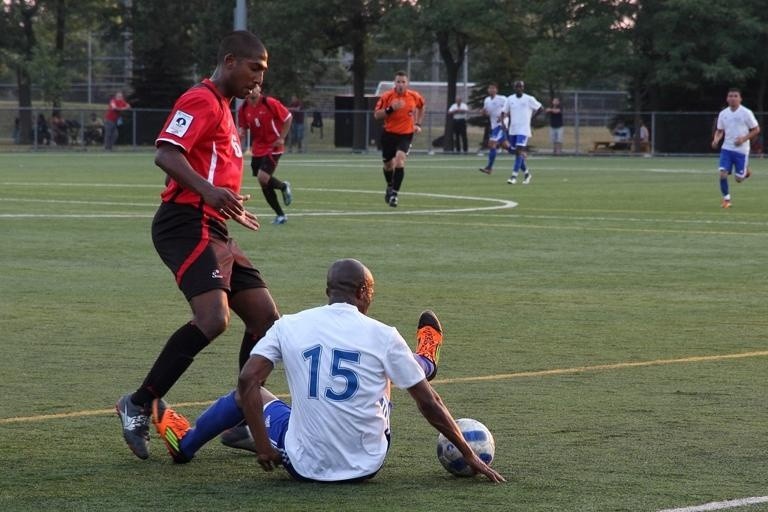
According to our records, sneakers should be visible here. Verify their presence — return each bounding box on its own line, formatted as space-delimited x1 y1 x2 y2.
389 195 398 206
722 199 732 208
153 399 192 463
480 167 491 174
522 173 531 183
415 309 443 381
115 394 152 459
507 177 517 184
222 426 259 452
271 216 288 224
282 181 291 204
385 189 392 203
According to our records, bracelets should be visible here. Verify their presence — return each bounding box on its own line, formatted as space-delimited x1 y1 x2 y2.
415 123 422 128
385 106 394 115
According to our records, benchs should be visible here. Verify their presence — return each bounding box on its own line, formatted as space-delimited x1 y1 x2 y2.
593 141 650 153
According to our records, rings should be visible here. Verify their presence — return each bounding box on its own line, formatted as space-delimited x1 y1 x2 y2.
218 208 226 215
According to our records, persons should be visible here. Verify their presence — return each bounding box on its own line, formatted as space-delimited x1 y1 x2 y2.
112 30 280 463
627 117 651 158
153 256 509 488
542 96 565 152
372 69 427 209
233 77 295 228
446 95 471 153
478 79 518 174
711 87 761 206
284 93 305 153
605 116 635 158
11 90 131 149
500 78 544 184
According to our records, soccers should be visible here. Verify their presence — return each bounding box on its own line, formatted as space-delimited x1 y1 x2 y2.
437 418 495 479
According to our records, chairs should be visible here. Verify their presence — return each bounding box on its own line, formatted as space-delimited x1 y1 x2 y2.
37 112 105 148
